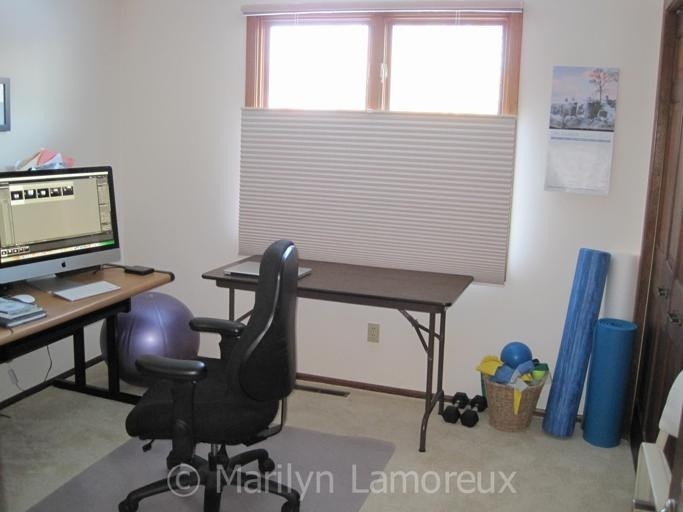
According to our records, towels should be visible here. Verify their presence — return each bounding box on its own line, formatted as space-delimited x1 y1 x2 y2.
484 375 547 432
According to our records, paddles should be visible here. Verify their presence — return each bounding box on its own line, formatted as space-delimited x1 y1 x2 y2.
27 426 396 512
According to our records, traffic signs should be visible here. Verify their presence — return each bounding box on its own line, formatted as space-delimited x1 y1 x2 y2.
368 324 378 342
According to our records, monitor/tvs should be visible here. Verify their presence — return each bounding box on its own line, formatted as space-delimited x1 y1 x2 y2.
0 297 44 320
0 296 47 329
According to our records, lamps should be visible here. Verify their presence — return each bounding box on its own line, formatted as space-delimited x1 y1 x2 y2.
117 239 300 511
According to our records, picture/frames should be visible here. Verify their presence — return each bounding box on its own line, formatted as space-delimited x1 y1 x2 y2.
202 254 473 451
0 265 175 405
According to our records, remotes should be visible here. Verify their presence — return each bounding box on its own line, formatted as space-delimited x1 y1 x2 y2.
13 293 37 304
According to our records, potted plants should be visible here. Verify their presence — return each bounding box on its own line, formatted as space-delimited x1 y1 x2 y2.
0 77 11 131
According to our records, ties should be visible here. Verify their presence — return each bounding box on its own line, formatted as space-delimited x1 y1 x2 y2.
0 165 120 295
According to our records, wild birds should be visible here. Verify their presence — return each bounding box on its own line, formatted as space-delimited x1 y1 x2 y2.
224 260 313 283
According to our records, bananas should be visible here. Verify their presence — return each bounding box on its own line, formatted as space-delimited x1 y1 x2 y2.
54 279 121 303
